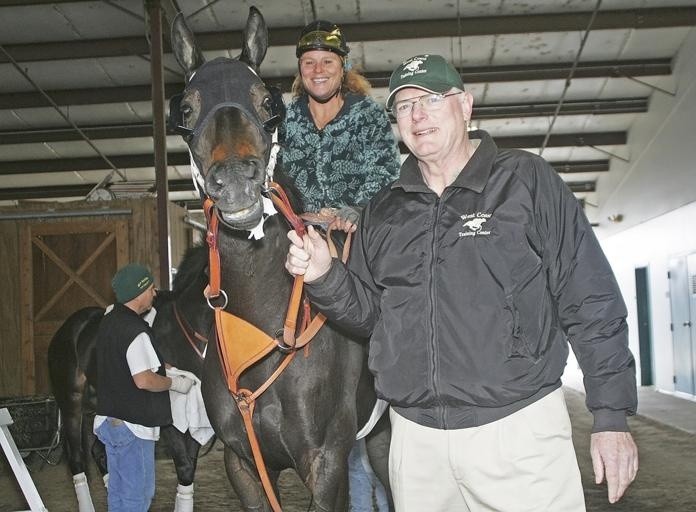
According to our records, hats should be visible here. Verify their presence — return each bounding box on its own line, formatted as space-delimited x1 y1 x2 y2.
386 55 465 111
111 264 153 303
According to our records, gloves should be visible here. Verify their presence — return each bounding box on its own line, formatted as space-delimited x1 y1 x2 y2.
169 376 195 394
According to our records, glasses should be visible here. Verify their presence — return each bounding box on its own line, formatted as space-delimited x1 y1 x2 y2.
297 30 345 53
391 92 464 118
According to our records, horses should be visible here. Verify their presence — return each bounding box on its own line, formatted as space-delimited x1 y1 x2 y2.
44 235 209 512
167 3 393 512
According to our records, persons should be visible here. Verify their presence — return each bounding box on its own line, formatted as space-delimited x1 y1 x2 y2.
92 263 195 512
271 20 400 234
284 54 642 510
349 445 390 512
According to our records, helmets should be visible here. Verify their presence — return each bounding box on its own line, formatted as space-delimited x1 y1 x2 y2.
296 19 346 56
